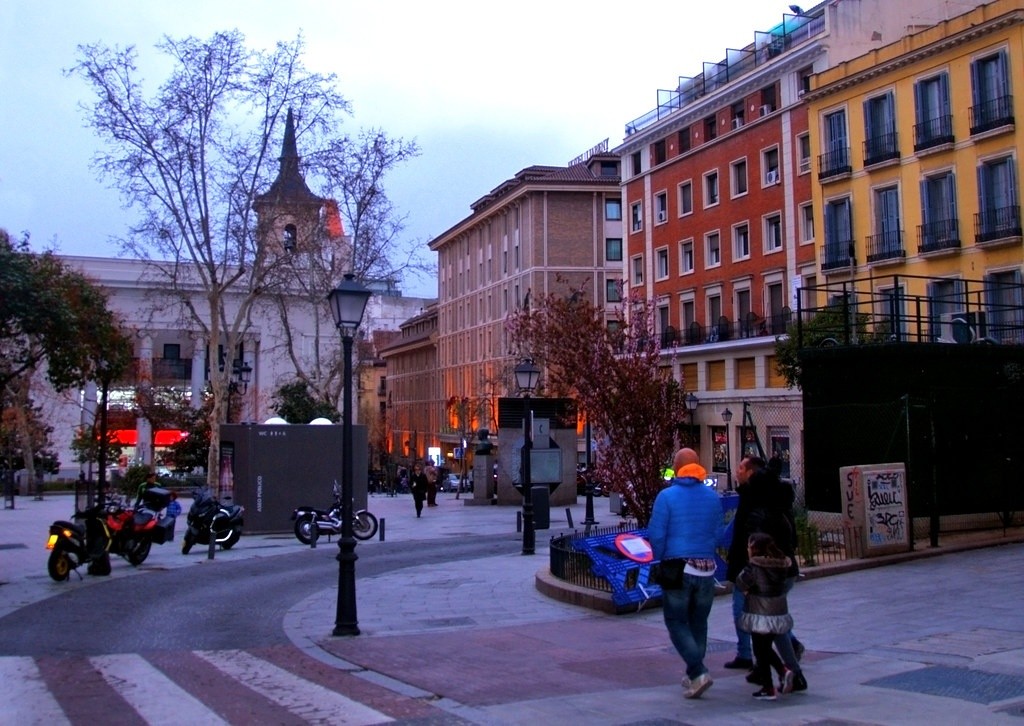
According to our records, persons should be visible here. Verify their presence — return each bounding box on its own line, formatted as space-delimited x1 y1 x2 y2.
134 473 161 507
721 454 808 700
475 428 493 455
647 447 725 698
467 465 474 493
409 463 428 518
426 480 438 506
166 493 181 541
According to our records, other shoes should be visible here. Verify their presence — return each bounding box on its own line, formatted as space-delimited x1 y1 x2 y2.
792 669 807 691
681 676 691 687
683 673 713 698
746 666 763 685
724 657 753 668
791 638 805 662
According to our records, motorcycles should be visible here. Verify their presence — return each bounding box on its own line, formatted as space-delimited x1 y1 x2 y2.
290 478 378 544
92 485 172 566
181 481 245 555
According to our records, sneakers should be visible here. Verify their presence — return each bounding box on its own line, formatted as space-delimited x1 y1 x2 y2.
779 668 793 694
752 686 776 699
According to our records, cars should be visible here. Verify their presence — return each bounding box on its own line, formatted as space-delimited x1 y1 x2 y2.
439 473 471 493
577 462 617 497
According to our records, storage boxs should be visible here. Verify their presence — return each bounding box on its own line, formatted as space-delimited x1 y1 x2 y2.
144 486 171 511
154 517 175 544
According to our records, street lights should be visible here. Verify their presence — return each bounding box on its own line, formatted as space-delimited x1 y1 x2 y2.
326 272 375 635
208 360 253 424
512 358 542 556
721 407 733 492
685 392 699 451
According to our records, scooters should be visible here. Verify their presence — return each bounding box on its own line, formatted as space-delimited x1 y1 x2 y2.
45 503 113 582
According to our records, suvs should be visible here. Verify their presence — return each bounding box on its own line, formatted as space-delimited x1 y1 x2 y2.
469 463 498 494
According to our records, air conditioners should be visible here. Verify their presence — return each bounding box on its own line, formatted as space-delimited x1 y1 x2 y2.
766 170 777 182
759 104 771 117
658 210 666 222
732 118 743 130
